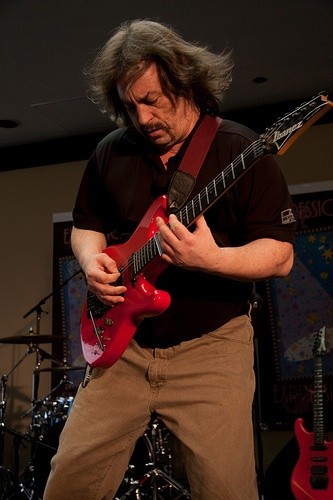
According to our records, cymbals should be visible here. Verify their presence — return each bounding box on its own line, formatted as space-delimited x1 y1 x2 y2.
0 334 67 344
37 366 84 372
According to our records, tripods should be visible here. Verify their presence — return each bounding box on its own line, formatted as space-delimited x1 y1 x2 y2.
113 418 191 500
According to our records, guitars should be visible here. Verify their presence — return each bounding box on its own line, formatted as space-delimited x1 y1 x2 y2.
80 93 333 367
290 326 333 500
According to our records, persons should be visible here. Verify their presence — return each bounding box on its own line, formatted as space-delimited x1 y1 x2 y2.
43 21 295 500
